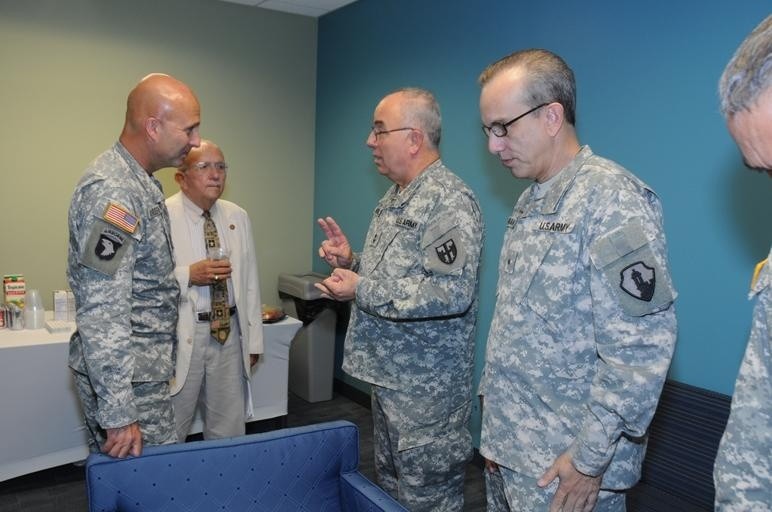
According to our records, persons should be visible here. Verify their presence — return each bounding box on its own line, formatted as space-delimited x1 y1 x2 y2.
472 47 678 512
66 70 202 458
311 84 486 511
160 139 266 445
713 14 772 512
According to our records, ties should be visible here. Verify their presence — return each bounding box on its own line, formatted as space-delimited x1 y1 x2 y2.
201 209 231 346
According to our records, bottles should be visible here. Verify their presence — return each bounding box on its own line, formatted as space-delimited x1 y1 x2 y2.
0 303 22 330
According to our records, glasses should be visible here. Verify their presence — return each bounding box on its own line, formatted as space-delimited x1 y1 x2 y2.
481 101 551 139
183 160 230 173
371 126 416 142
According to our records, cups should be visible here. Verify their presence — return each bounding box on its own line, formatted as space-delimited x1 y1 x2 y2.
23 288 46 331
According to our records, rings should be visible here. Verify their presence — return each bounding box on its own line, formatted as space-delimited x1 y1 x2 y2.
214 275 219 282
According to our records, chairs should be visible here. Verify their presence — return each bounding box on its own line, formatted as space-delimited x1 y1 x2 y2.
82 416 410 511
623 372 735 512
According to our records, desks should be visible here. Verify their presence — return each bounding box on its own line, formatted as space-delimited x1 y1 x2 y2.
0 287 304 492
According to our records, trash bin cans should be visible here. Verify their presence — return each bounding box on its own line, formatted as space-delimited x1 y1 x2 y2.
278 272 336 403
